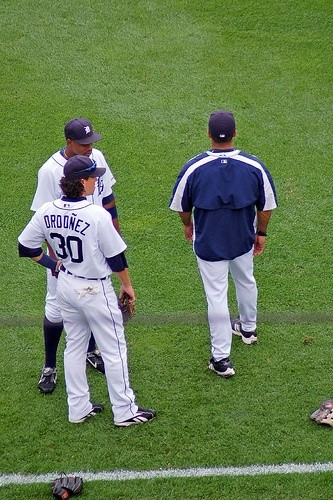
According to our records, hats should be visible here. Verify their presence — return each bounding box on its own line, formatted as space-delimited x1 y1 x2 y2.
208 111 235 134
64 155 106 177
64 119 102 144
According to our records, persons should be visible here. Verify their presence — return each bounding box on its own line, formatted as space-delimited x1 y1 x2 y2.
17 155 157 426
29 118 121 393
169 110 278 378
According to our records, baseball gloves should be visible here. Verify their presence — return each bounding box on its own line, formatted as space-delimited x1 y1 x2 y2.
112 293 137 323
308 397 333 428
49 473 83 500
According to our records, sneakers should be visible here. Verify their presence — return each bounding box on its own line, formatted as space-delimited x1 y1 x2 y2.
69 404 104 423
207 357 235 377
113 409 154 424
37 370 57 395
232 320 257 343
86 351 106 374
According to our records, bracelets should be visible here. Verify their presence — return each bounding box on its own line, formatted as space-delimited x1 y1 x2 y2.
257 228 267 236
